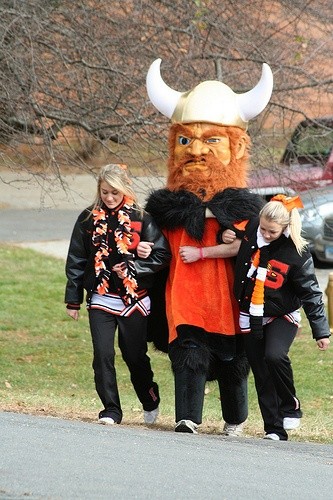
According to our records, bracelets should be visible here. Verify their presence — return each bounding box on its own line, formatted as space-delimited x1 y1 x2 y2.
200 247 203 260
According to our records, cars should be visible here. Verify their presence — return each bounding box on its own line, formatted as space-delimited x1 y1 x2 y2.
248 117 333 268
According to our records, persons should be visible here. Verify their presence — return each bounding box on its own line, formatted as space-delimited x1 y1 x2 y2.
138 58 273 438
217 194 331 440
64 164 171 425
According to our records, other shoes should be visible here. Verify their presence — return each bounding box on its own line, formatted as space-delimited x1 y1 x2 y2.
143 406 160 427
174 419 199 434
96 417 118 425
222 419 249 437
263 433 280 441
283 417 300 431
